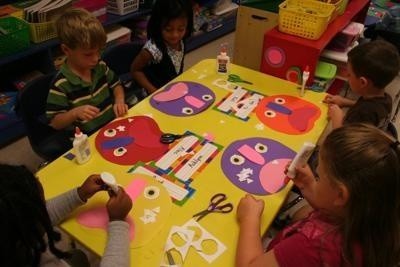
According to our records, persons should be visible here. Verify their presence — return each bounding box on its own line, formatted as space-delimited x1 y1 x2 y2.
234 122 399 267
129 0 190 100
0 163 133 267
308 38 399 180
44 7 129 150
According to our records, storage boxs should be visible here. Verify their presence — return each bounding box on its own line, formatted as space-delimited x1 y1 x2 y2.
88 0 140 55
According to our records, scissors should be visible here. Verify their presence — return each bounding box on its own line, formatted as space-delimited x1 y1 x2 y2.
194 193 233 221
160 133 187 144
227 74 253 85
297 85 324 93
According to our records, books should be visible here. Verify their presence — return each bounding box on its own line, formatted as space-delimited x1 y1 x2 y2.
22 0 73 23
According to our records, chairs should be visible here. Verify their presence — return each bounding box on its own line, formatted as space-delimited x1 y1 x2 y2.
13 40 145 164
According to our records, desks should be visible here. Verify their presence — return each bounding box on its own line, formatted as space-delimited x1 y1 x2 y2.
37 56 337 267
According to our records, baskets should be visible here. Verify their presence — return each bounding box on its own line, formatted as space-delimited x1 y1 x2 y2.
106 0 140 16
278 0 347 40
0 10 58 58
